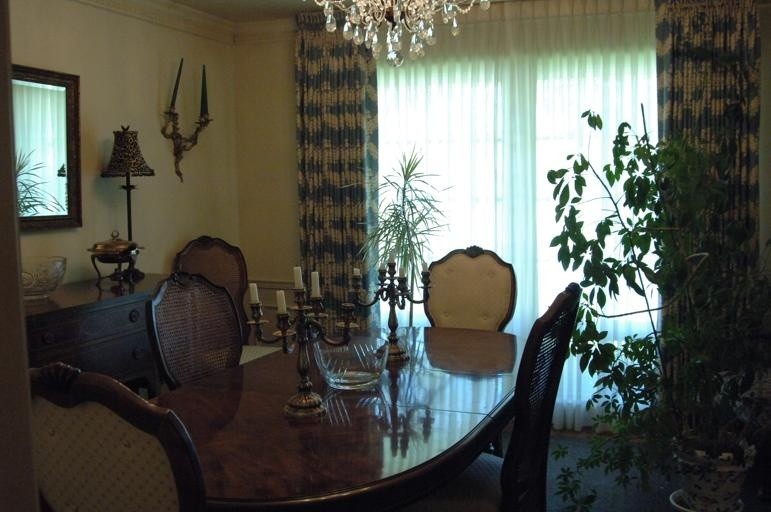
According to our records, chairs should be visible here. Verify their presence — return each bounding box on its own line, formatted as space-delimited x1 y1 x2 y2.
147 274 243 389
422 245 515 332
396 281 582 510
28 361 207 511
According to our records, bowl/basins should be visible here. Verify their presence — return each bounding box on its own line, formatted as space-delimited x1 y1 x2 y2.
22 256 67 300
312 333 389 390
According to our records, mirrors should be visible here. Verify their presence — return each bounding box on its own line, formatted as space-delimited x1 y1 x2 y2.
10 63 83 231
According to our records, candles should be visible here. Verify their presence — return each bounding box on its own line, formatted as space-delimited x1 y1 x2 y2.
353 253 406 279
311 272 319 297
294 267 303 288
249 283 259 302
276 290 286 313
422 263 427 272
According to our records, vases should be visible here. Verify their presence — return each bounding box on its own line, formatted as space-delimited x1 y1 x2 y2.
671 428 752 511
667 488 745 511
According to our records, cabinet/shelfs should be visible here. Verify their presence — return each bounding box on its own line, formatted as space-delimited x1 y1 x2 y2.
24 274 164 393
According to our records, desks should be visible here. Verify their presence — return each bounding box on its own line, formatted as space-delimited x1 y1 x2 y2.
148 327 528 512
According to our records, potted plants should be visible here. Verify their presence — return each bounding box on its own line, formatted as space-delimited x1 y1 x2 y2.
544 109 768 511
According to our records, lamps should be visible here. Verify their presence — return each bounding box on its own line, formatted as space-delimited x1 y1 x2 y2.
314 0 489 70
101 125 155 280
